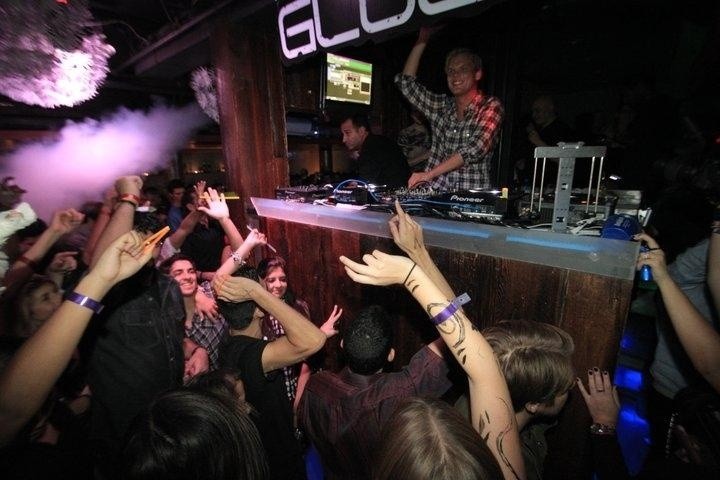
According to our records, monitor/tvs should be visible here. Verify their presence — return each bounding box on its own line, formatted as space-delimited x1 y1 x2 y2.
321 51 374 116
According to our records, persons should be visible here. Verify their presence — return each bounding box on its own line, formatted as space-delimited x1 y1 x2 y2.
1 167 719 480
339 109 404 182
401 29 501 200
396 105 434 173
522 91 571 188
